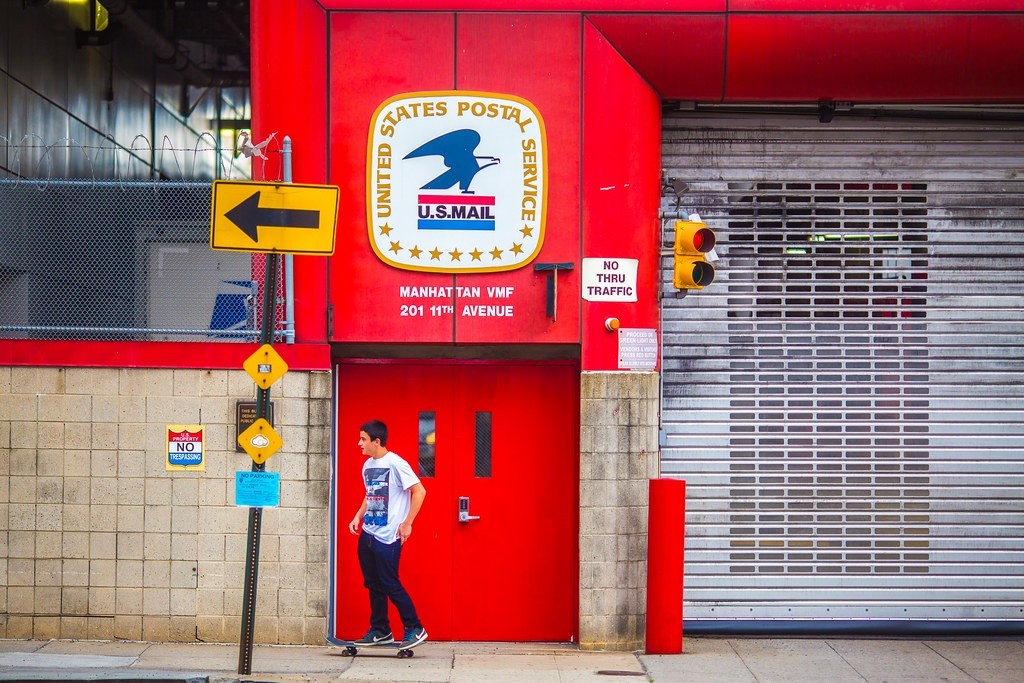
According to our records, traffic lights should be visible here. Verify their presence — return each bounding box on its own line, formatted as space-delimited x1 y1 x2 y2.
673 221 716 294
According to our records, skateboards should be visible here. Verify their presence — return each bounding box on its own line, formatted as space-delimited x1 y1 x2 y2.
325 636 427 658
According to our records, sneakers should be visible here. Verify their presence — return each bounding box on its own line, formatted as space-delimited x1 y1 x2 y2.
354 627 395 646
399 627 428 650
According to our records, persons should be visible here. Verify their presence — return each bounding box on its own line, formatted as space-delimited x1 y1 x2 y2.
349 421 428 650
